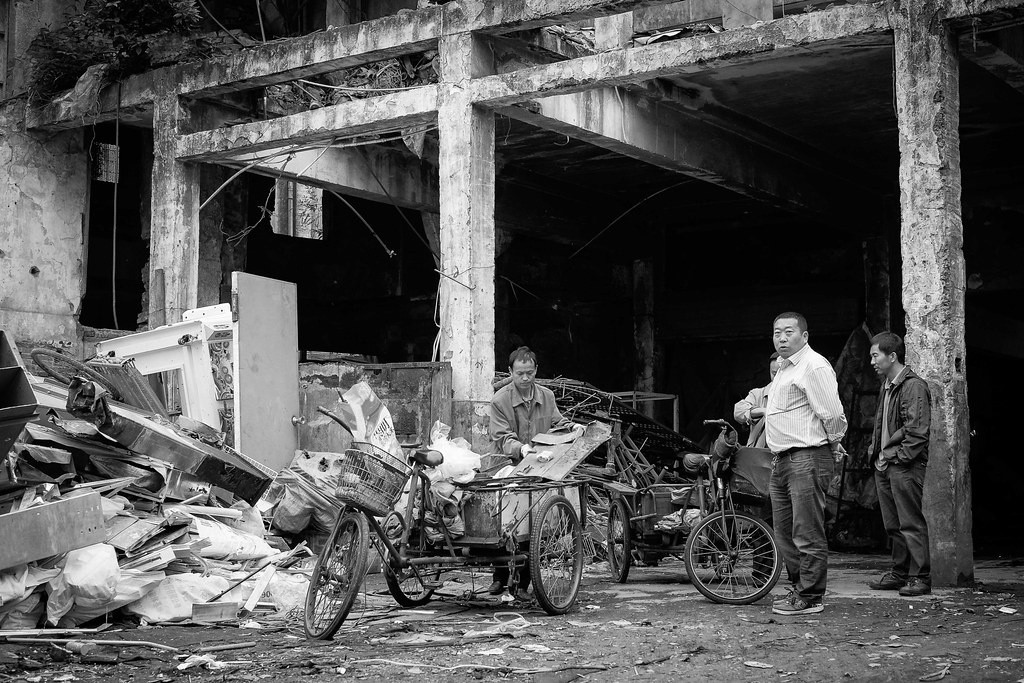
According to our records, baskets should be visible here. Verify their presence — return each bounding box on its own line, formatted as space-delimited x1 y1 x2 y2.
335 442 413 517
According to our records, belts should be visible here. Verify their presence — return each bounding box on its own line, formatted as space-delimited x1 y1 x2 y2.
773 446 802 462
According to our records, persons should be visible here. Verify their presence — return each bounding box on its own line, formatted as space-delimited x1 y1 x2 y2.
766 312 848 614
734 352 780 588
488 346 587 602
866 332 931 596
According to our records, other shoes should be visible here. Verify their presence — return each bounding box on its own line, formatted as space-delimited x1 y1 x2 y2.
509 588 532 601
753 578 767 588
489 581 504 595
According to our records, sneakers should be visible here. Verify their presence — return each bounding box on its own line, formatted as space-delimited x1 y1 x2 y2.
869 572 909 590
770 586 824 615
899 578 931 595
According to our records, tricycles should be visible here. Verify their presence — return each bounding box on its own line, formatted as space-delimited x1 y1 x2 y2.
301 403 591 642
603 417 782 606
